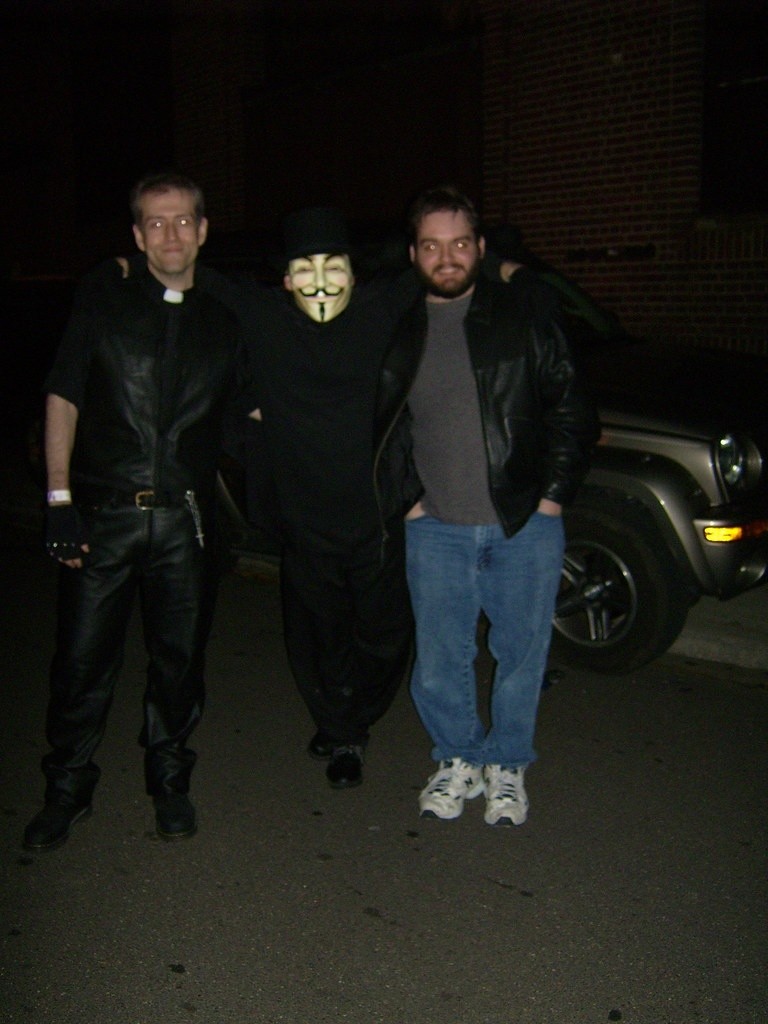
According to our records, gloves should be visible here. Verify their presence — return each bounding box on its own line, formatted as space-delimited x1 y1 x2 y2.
510 265 560 333
89 258 124 288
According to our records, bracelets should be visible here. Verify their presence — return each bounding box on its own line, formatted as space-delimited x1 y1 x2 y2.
47 489 71 503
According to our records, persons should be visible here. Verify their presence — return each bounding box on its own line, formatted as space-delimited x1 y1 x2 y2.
371 187 602 826
86 206 545 791
22 171 250 855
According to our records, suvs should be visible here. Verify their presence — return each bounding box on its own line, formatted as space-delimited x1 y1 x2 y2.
1 263 768 680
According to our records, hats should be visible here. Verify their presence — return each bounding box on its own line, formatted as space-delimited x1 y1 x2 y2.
270 208 362 259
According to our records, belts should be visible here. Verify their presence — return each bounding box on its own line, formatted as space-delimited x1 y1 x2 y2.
74 484 186 510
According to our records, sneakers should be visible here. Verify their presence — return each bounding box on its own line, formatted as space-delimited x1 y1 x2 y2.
483 763 528 825
417 757 487 819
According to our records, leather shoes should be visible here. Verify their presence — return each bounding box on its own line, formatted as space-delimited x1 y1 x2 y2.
310 727 338 759
22 805 92 850
326 744 362 789
153 799 198 841
